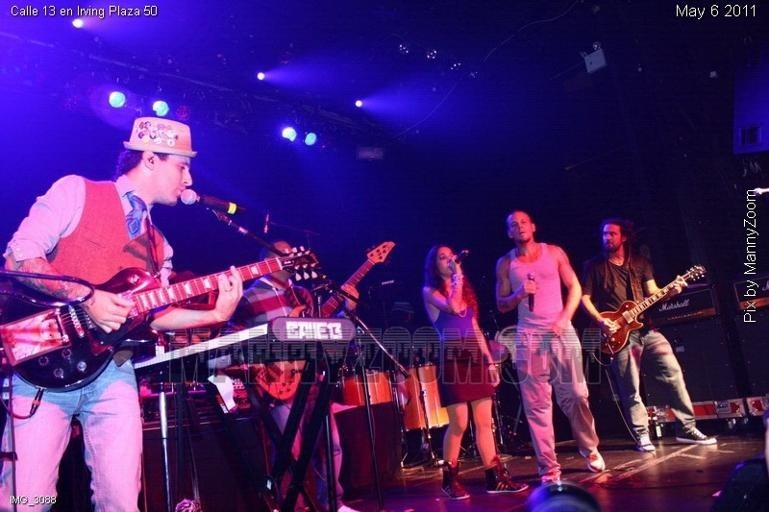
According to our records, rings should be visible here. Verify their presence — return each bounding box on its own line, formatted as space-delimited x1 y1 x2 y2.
225 287 233 293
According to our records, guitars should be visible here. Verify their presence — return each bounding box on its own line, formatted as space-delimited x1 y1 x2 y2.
254 241 396 400
1 245 320 393
589 264 707 355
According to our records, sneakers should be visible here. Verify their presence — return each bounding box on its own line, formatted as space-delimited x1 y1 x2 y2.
635 432 656 452
337 505 357 512
542 473 562 486
485 456 529 494
676 426 717 445
441 462 470 500
580 449 605 472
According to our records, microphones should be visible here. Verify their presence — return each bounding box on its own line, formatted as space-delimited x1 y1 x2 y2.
527 271 538 313
180 189 248 218
454 250 473 264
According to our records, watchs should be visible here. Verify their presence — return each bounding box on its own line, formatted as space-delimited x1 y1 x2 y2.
487 363 500 368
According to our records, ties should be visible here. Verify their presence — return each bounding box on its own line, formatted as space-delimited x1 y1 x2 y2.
123 192 146 238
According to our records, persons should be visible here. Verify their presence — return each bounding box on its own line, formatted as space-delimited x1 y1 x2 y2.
496 212 606 491
231 240 359 512
582 222 718 452
2 118 243 512
421 247 529 500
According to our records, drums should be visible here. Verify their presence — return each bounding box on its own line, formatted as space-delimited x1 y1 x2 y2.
336 366 395 410
391 363 449 431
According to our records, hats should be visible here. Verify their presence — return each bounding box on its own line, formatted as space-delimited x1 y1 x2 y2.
123 116 197 158
